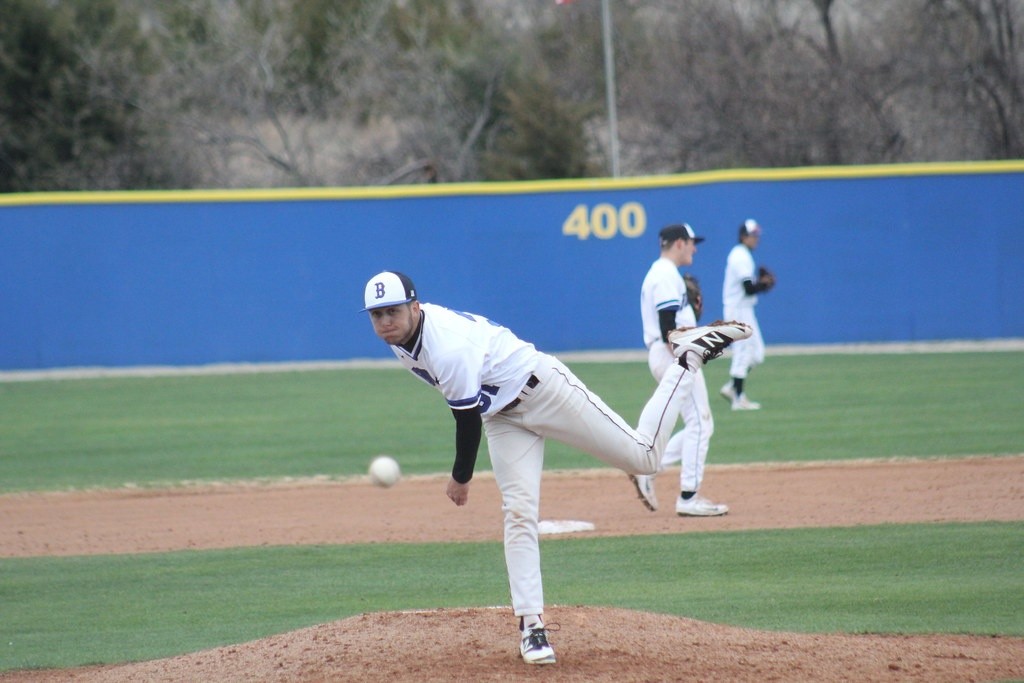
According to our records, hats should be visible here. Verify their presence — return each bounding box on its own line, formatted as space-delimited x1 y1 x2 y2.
357 271 417 313
739 219 763 236
658 222 706 244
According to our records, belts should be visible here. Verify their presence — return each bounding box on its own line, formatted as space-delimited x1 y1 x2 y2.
501 374 540 412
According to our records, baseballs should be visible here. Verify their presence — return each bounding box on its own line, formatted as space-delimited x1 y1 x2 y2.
369 455 400 488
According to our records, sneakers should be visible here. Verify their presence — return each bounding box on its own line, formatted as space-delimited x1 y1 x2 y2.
720 379 734 405
676 490 728 517
665 320 753 372
629 474 659 512
731 394 762 411
519 616 562 665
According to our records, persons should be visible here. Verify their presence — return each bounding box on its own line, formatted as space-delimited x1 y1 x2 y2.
720 218 775 410
359 270 752 666
628 224 729 516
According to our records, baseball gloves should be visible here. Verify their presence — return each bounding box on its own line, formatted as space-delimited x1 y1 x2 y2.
759 267 775 288
683 272 703 322
